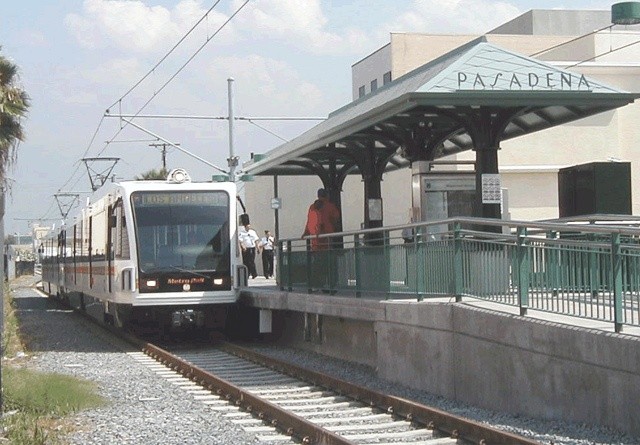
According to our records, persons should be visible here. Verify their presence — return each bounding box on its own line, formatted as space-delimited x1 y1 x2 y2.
177 231 211 268
259 230 275 279
238 224 260 279
402 208 420 285
301 189 341 293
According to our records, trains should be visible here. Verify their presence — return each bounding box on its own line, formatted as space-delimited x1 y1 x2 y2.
38 168 250 332
